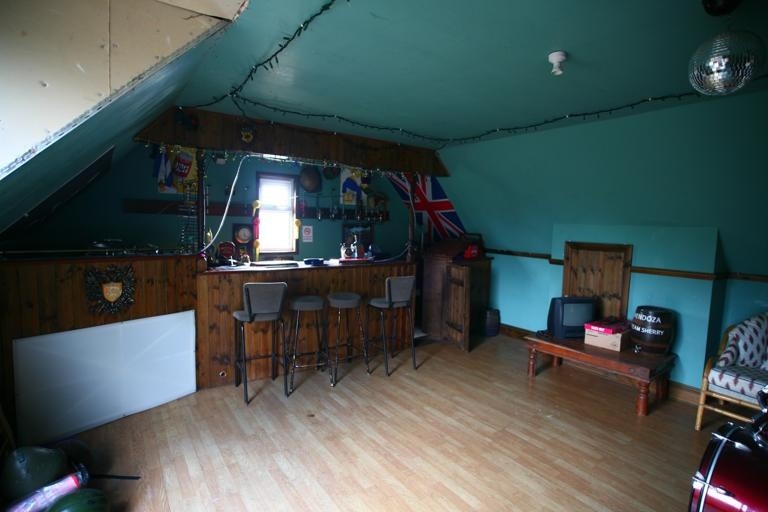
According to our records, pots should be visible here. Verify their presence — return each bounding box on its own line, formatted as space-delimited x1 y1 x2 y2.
323 167 340 179
299 165 322 193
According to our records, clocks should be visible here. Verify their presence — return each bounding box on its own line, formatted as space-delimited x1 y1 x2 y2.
235 225 253 244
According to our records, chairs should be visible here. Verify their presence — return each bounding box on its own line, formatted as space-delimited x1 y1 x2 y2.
233 282 288 404
694 312 768 431
364 276 416 377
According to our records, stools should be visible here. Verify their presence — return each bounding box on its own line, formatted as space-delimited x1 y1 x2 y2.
286 295 334 393
320 291 371 376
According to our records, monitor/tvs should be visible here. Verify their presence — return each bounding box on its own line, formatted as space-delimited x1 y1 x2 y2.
548 297 600 338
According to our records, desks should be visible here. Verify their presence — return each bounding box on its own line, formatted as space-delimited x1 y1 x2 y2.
523 330 681 417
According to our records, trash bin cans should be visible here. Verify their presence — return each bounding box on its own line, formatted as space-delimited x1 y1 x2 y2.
480 308 500 337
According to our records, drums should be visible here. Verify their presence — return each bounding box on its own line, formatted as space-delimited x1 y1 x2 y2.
746 408 768 449
687 422 768 512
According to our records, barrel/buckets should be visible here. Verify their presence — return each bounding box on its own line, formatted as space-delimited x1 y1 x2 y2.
631 306 678 359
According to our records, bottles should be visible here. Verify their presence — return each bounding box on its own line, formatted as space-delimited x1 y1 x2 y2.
237 246 250 266
339 242 352 258
351 234 364 259
